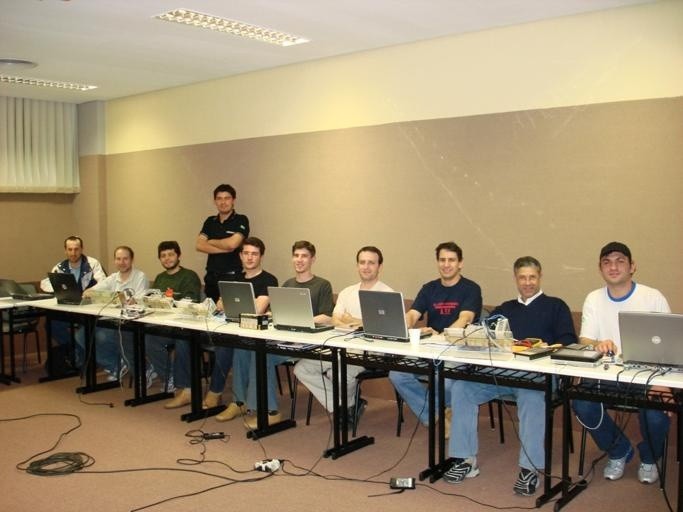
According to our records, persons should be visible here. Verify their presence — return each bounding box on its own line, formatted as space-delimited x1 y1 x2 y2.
214 240 332 427
441 257 577 497
572 242 677 483
74 245 149 381
388 242 482 438
162 237 278 410
39 235 107 343
194 183 249 303
142 241 200 395
292 246 396 430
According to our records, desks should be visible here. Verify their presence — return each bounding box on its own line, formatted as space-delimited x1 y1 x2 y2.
0 294 683 512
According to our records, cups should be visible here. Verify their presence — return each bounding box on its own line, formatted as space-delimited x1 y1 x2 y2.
408 328 422 347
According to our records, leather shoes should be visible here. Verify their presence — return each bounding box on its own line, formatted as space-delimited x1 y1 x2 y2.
164 387 191 408
243 410 282 429
202 390 223 410
216 400 247 421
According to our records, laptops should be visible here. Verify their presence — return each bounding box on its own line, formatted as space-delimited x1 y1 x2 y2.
618 311 683 372
267 286 335 333
359 290 432 342
48 272 93 304
218 281 272 324
0 279 53 301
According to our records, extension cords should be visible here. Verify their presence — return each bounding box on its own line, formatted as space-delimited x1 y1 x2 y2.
254 458 280 472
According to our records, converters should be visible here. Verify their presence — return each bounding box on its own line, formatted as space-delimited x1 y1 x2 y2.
203 432 224 439
391 478 415 489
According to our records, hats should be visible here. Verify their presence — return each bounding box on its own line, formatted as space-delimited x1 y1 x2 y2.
600 242 631 260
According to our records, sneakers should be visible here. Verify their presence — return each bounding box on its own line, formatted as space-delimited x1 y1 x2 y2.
603 446 634 481
638 458 662 484
108 365 128 382
340 398 367 432
145 364 158 389
512 469 540 497
160 374 177 393
443 462 480 484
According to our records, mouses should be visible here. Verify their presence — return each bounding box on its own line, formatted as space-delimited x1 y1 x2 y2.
605 349 615 357
